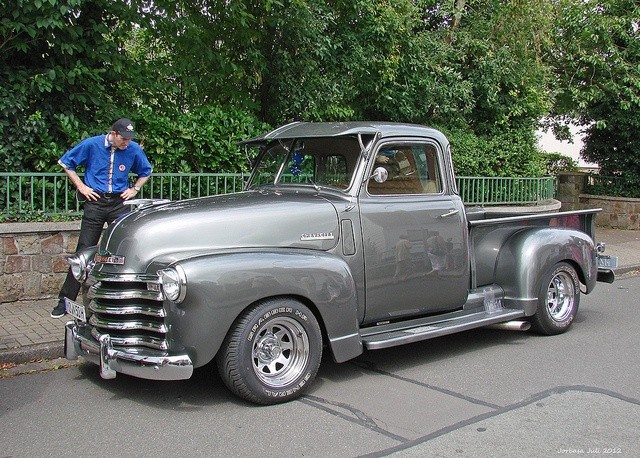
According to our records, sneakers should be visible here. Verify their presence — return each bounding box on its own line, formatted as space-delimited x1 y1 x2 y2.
51 300 68 318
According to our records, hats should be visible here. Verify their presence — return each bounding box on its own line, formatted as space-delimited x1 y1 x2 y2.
113 118 136 139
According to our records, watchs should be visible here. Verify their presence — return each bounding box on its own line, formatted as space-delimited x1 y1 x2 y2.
132 186 141 191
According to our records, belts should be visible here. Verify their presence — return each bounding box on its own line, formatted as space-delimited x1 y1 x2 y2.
98 192 122 198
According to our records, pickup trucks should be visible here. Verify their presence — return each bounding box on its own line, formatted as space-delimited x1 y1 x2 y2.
63 119 619 405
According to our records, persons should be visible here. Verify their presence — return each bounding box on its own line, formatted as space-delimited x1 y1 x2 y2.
51 118 153 318
